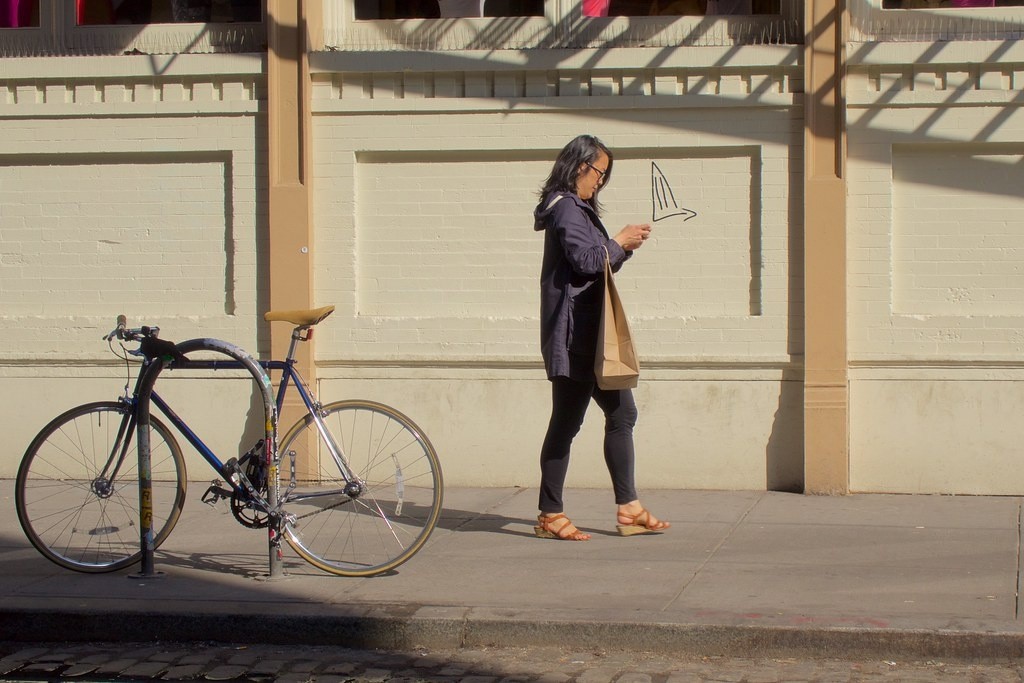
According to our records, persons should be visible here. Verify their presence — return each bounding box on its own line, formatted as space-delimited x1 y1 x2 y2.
534 135 669 541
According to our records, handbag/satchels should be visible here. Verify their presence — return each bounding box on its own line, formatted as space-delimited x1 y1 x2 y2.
594 245 640 391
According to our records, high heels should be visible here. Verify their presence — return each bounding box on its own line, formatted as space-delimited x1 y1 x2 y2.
534 514 590 541
615 509 671 536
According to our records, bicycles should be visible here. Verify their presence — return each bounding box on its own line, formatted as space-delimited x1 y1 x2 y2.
14 305 444 577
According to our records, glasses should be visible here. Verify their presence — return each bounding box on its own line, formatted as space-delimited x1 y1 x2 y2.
584 160 607 184
